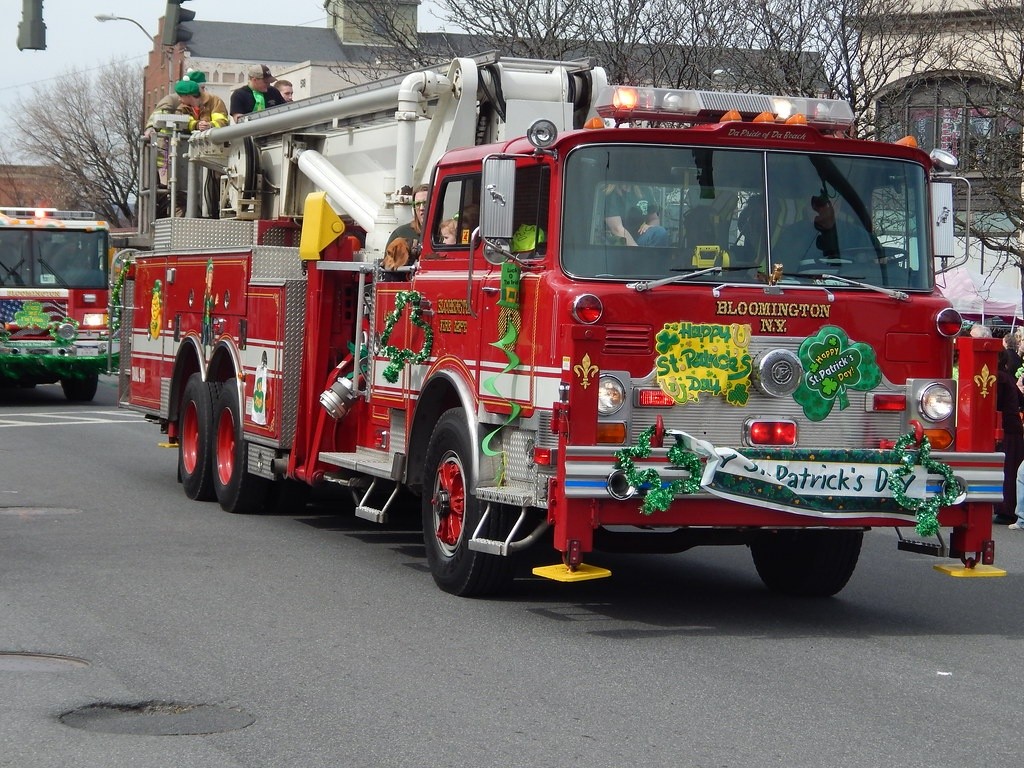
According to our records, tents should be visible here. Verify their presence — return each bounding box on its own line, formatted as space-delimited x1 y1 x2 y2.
935 251 1023 335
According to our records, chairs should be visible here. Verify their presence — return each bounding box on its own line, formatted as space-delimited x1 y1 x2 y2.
753 197 854 274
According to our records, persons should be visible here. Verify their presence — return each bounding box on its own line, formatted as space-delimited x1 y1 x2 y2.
174 81 230 135
438 219 458 244
384 184 444 266
50 239 87 270
274 80 293 102
144 71 206 185
604 182 668 248
769 181 882 284
230 64 287 124
989 327 1024 530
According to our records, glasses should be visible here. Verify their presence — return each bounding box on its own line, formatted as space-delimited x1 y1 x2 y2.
412 201 427 208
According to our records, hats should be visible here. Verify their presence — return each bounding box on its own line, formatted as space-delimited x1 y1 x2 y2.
174 76 200 96
248 64 278 83
807 180 842 208
186 68 206 84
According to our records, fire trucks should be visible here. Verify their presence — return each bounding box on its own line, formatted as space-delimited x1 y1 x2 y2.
107 51 1007 601
0 207 119 405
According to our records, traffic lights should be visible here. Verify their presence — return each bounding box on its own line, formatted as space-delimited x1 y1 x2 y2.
161 0 196 47
